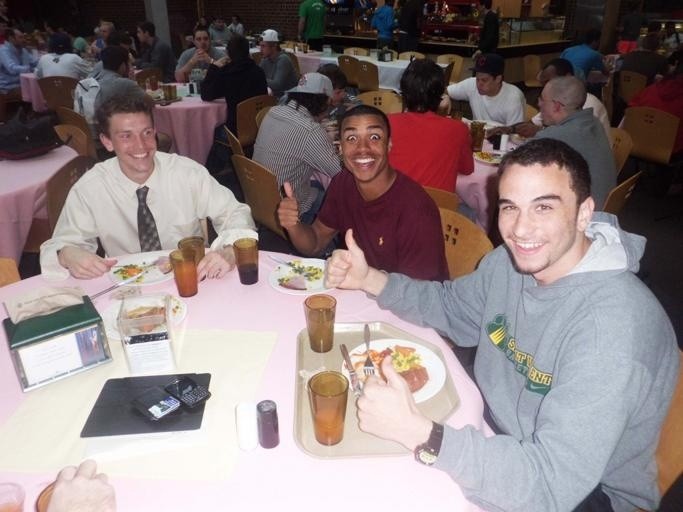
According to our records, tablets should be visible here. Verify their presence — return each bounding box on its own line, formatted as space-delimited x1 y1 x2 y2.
10 320 115 392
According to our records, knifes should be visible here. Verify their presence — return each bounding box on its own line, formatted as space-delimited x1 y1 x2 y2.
339 342 362 394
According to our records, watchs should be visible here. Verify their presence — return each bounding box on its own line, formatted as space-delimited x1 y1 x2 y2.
413 420 444 467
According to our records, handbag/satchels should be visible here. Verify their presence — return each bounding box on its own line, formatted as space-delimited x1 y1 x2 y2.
0 106 63 160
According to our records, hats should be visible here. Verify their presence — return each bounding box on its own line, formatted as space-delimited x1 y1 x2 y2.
259 29 280 43
284 72 334 98
468 53 505 74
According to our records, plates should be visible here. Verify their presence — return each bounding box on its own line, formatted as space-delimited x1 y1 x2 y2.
267 258 338 295
341 337 447 404
102 250 175 284
99 291 188 340
473 151 504 165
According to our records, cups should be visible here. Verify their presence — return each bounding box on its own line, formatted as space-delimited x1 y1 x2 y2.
143 68 207 99
471 129 484 152
303 292 338 352
451 110 462 119
176 236 205 265
308 370 349 445
233 237 260 285
169 248 199 300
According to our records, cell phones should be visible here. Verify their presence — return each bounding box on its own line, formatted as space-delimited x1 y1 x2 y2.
164 375 211 408
130 386 182 423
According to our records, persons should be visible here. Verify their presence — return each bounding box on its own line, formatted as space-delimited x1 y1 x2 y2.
276 103 451 283
37 96 260 281
321 137 679 511
1 1 682 228
46 458 117 512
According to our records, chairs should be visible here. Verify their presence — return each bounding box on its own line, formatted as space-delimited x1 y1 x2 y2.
36 75 78 108
344 48 368 56
354 60 378 91
620 106 681 171
618 71 647 105
45 156 102 233
355 89 402 119
606 126 634 174
436 55 464 84
233 154 287 245
56 106 91 141
55 124 88 158
423 187 459 215
602 78 615 123
438 209 498 280
399 51 426 62
237 95 275 148
338 54 359 85
222 124 243 162
656 348 683 504
603 171 642 216
523 55 543 87
0 256 23 287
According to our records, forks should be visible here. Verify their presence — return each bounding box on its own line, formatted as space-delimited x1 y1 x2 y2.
363 321 376 378
